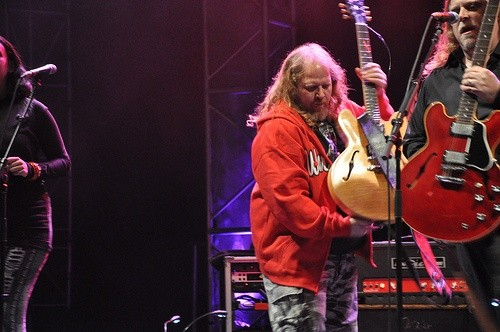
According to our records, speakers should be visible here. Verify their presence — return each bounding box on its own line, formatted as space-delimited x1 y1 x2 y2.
356 242 482 332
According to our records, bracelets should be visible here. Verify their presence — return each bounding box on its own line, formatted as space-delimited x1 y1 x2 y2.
26 161 41 181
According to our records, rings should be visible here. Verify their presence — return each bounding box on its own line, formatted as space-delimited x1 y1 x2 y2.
467 73 470 79
467 79 472 86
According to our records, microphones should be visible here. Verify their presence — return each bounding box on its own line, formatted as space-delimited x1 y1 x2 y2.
20 64 57 78
432 12 459 25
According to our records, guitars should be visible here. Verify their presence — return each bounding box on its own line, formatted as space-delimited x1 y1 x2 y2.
401 0 500 242
327 1 410 225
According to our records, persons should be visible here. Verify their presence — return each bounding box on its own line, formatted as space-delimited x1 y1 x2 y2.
402 1 500 331
250 43 396 332
0 36 73 332
